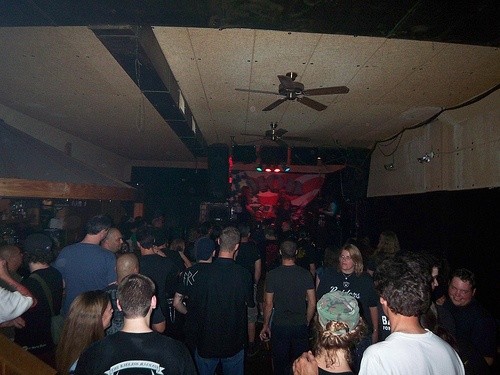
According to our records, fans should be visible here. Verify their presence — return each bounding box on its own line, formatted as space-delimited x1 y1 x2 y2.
237 122 310 150
235 73 349 112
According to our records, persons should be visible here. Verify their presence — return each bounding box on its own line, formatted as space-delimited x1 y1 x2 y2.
0 186 496 375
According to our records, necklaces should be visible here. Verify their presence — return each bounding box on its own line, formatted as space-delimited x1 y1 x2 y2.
340 269 352 279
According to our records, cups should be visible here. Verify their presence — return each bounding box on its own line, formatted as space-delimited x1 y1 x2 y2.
263 337 271 352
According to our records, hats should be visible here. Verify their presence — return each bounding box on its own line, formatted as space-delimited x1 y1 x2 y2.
24 233 52 253
316 291 360 331
194 236 216 259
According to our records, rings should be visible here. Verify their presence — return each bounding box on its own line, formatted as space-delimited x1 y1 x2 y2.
259 334 262 336
